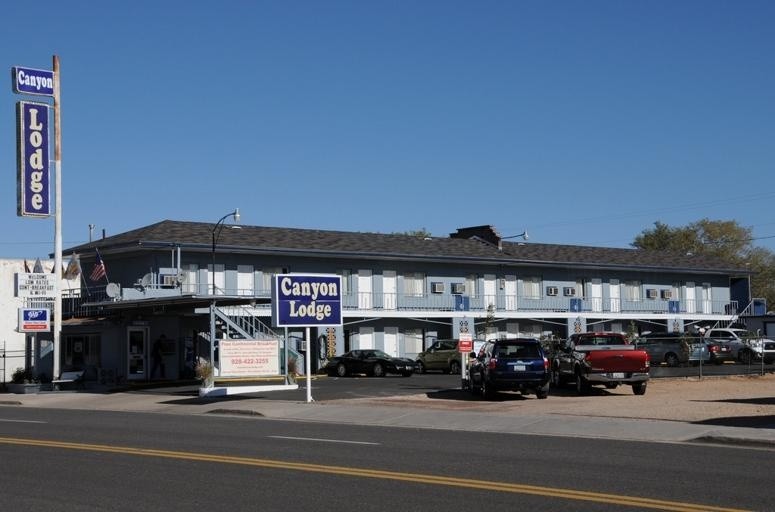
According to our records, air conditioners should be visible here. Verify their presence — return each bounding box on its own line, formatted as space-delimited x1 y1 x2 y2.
646 289 658 298
563 287 576 296
298 340 307 351
661 290 672 298
546 287 559 296
162 275 178 286
453 283 465 293
431 283 444 293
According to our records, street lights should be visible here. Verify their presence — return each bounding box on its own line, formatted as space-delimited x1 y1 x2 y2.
209 205 242 388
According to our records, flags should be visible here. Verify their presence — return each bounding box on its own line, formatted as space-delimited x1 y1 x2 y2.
89 252 106 281
24 259 31 279
64 253 82 280
50 262 65 281
32 258 44 279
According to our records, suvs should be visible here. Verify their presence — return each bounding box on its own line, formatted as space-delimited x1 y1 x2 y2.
469 336 556 402
414 338 488 375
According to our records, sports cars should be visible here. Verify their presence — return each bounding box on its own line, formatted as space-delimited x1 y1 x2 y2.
323 347 419 379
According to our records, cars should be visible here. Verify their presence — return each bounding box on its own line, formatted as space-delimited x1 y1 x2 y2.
541 326 775 385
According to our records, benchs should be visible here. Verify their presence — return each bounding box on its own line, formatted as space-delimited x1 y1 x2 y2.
51 369 87 392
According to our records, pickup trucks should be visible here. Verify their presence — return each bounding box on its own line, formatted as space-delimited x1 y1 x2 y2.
550 331 651 395
